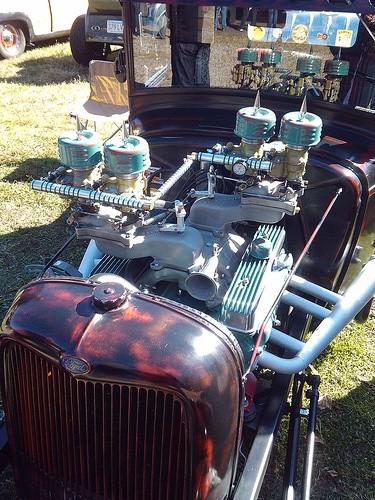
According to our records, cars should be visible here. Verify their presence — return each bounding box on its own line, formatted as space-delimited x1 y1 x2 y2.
0 0 89 57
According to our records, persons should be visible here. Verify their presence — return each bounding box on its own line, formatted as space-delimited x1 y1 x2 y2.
239 8 258 32
267 9 278 28
132 3 168 40
215 6 227 32
166 4 215 87
